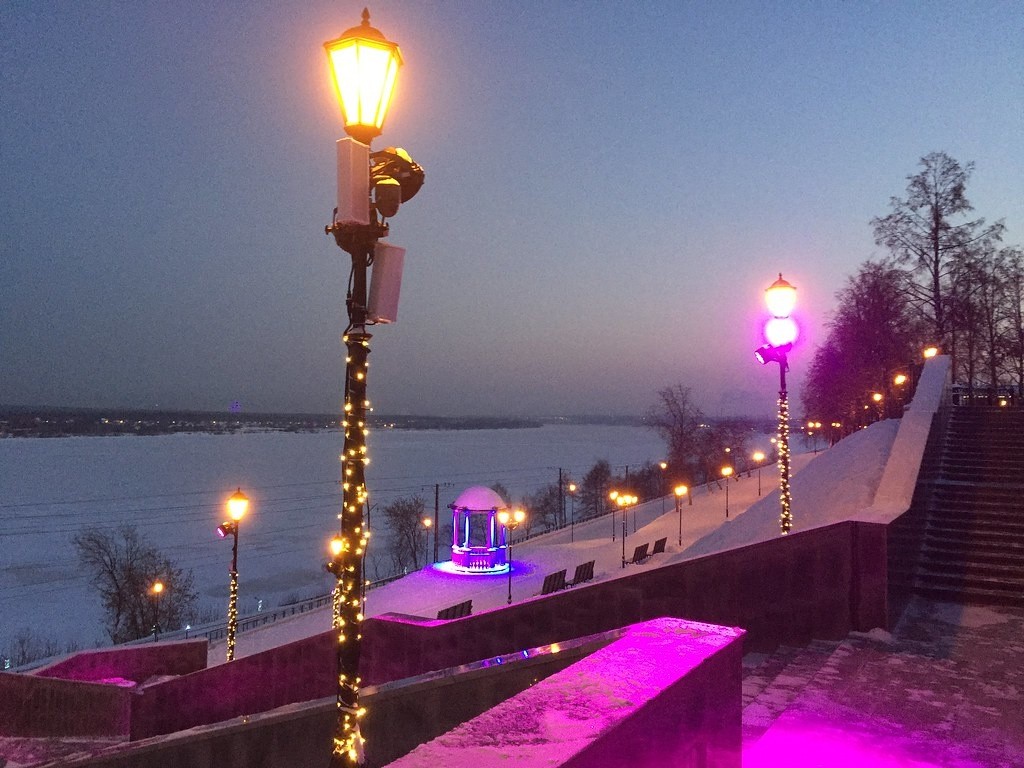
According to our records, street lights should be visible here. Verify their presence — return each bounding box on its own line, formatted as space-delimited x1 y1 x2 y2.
151 581 164 642
217 487 252 663
721 467 734 518
632 496 638 532
610 492 617 542
660 463 667 515
569 484 576 543
807 422 822 456
830 422 840 446
751 453 764 498
421 518 432 569
673 486 689 547
495 509 527 605
756 272 801 534
315 7 426 768
618 495 633 568
